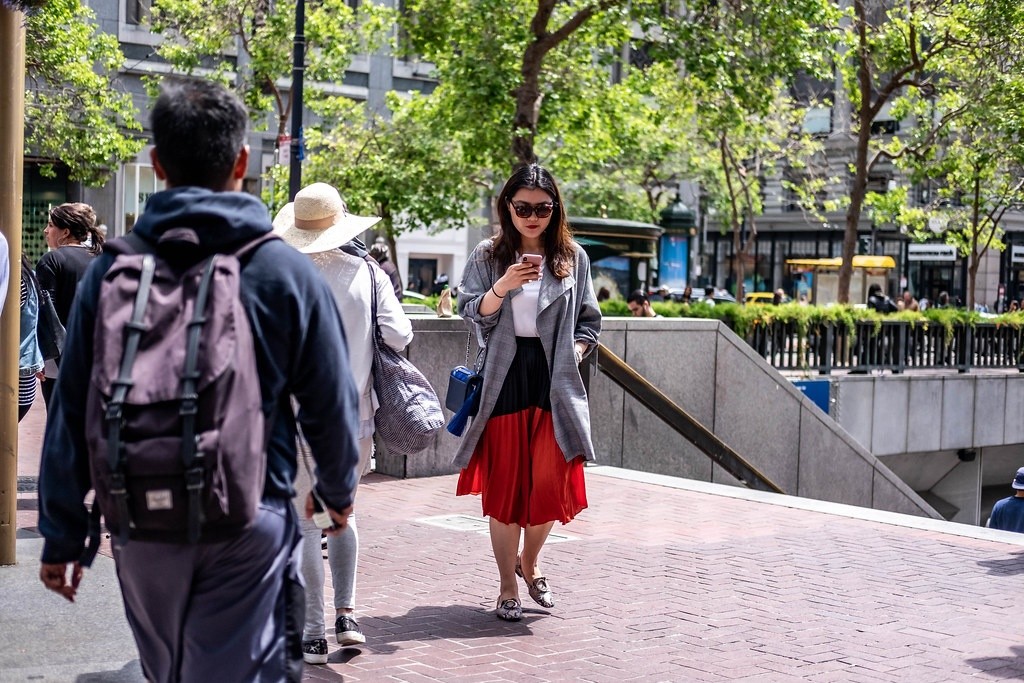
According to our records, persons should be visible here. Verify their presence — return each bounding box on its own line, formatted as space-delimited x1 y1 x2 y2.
989 467 1024 533
680 287 694 303
370 243 402 301
1017 299 1024 311
1010 300 1019 312
627 289 663 317
33 201 107 410
37 75 360 683
772 288 809 352
897 291 950 312
863 284 898 374
406 282 414 291
272 182 414 664
492 286 505 298
698 287 715 307
436 274 448 295
18 251 45 423
455 163 602 622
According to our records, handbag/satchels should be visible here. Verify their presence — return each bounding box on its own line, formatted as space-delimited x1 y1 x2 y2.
365 262 445 456
446 365 484 417
888 300 898 313
23 262 67 361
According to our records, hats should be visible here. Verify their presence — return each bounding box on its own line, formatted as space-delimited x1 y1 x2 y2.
272 181 382 253
1011 467 1024 490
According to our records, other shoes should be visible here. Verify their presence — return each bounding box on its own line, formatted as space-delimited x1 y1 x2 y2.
497 592 522 621
517 555 554 608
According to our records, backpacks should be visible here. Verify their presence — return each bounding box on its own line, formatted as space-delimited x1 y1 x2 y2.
85 228 282 540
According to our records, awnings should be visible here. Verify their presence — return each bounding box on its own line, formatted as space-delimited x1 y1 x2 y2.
786 255 895 272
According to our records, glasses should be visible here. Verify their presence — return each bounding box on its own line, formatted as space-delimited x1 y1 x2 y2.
509 198 555 218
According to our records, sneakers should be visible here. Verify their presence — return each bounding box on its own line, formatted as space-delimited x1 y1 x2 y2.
334 617 366 645
301 638 328 665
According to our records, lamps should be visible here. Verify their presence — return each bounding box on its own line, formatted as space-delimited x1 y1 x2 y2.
956 448 976 461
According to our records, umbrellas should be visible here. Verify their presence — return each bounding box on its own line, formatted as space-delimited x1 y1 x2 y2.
573 236 619 262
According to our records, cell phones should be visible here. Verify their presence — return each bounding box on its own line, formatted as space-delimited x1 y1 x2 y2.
521 253 542 281
